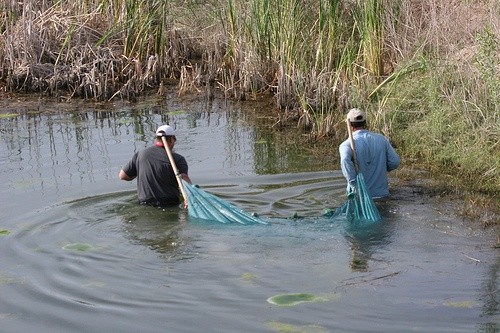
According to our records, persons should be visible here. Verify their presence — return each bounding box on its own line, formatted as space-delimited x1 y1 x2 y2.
339 108 401 199
120 125 192 209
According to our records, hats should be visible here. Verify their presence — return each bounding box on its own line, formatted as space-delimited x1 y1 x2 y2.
156 124 175 136
345 108 365 122
